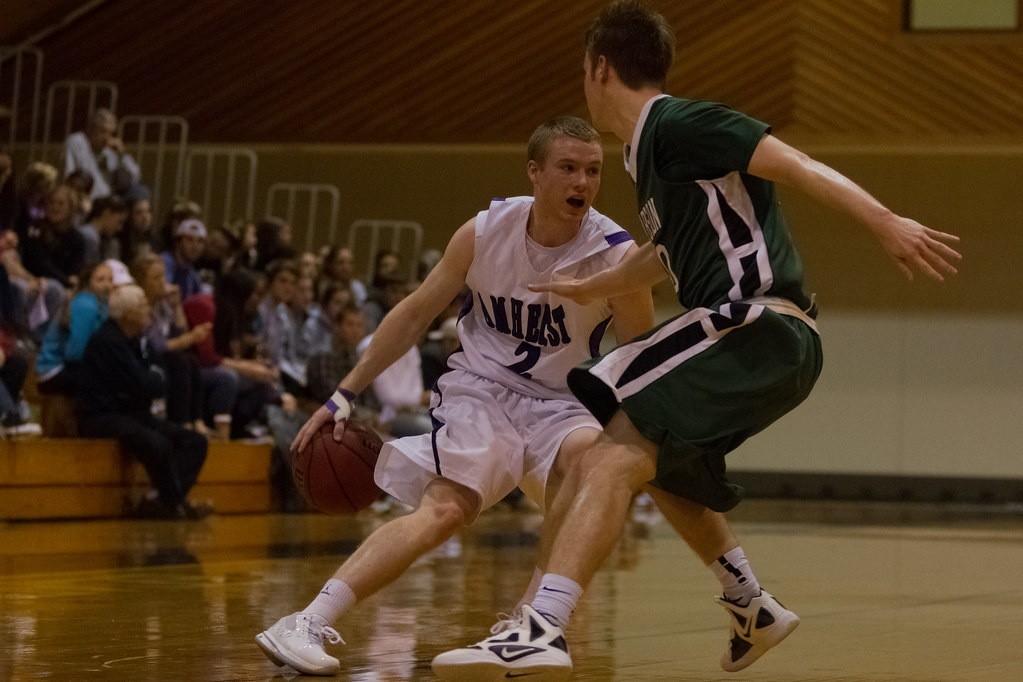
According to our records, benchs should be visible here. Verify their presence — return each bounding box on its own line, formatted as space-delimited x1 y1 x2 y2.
0 367 280 522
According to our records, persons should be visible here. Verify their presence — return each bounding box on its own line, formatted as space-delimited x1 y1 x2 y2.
253 116 656 676
0 106 463 557
431 0 964 682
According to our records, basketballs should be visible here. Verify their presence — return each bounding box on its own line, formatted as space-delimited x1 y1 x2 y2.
291 418 385 515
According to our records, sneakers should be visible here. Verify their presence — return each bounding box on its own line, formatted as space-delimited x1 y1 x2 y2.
713 587 800 672
254 612 344 675
430 604 572 682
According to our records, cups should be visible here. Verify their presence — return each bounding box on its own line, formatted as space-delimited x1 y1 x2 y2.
215 414 231 441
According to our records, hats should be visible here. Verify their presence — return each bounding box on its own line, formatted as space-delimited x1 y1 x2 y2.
105 259 134 287
176 219 207 237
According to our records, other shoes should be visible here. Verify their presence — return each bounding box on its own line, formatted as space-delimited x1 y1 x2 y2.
1 415 42 438
227 427 274 446
182 500 214 521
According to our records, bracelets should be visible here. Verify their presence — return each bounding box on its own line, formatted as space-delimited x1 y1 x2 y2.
325 387 356 422
